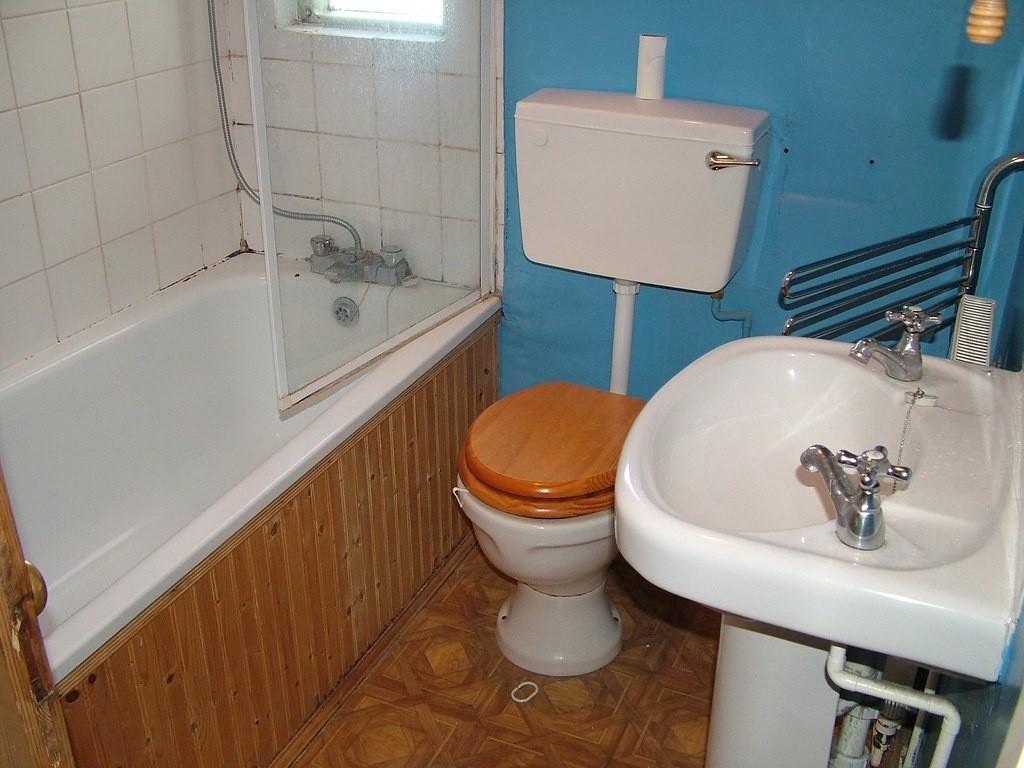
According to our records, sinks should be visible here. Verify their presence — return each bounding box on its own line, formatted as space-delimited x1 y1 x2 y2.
612 337 992 681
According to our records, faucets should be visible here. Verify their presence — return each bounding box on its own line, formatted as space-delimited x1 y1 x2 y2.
322 261 366 284
801 443 913 550
849 301 944 382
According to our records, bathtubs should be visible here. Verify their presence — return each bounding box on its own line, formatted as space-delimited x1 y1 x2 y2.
1 250 503 767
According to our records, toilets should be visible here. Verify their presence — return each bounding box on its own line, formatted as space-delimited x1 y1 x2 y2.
457 87 773 677
